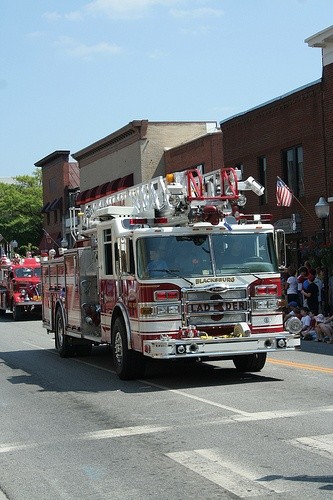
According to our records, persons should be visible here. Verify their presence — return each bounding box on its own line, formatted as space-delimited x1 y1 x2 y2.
218 240 248 264
145 245 197 278
280 261 333 345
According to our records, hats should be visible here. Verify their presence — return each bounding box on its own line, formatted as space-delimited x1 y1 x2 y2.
304 274 315 281
288 301 297 307
313 314 326 322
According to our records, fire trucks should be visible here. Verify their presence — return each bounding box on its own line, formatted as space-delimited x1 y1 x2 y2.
0 257 42 321
41 164 301 381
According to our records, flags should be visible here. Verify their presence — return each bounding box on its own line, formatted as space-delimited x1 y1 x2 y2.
44 231 52 244
276 180 293 206
0 234 3 242
